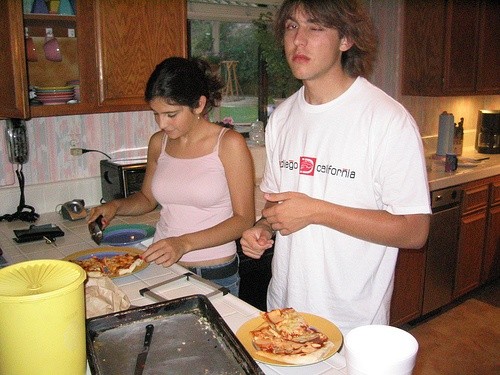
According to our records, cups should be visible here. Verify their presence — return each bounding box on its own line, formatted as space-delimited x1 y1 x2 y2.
23 0 75 15
343 324 419 375
445 153 458 173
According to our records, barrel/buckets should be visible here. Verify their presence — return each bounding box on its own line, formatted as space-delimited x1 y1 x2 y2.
0 258 87 375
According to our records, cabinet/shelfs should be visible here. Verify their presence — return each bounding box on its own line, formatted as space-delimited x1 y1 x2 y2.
389 175 500 327
0 0 188 120
403 0 500 97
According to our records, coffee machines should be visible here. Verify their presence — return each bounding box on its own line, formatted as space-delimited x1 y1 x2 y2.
475 109 500 153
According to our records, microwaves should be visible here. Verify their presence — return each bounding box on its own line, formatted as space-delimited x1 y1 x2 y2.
99 156 148 201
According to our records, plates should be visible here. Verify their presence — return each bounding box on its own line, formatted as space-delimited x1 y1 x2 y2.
33 85 76 105
61 247 149 279
236 311 343 367
440 157 484 167
101 224 156 247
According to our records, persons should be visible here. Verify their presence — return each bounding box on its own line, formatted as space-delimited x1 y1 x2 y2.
240 0 433 334
87 57 255 298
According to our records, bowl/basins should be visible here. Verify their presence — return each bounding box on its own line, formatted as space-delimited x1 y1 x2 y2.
43 33 61 61
25 37 38 62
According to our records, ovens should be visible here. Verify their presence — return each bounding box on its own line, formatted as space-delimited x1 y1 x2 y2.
232 233 276 311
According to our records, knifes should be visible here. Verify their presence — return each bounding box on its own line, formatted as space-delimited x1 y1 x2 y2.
454 117 464 139
134 324 154 375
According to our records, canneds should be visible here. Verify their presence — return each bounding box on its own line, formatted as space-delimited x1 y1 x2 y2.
445 154 457 172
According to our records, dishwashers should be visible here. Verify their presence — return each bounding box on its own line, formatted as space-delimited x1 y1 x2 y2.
421 187 462 314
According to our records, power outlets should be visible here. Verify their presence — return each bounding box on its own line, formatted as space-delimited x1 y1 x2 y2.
67 134 83 160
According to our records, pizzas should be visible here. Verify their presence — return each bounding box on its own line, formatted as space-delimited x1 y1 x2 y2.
251 307 333 364
66 253 144 277
